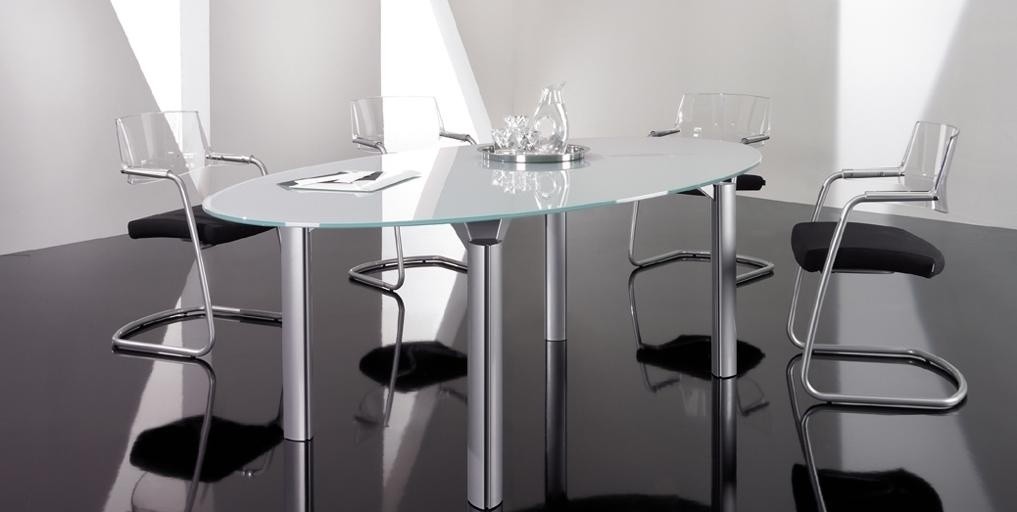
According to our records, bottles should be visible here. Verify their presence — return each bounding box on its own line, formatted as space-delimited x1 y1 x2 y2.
529 172 569 209
530 77 567 152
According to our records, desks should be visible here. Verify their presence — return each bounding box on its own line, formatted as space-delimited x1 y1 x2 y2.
201 136 763 511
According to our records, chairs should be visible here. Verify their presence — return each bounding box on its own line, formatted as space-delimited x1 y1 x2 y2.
785 353 942 512
350 266 471 430
628 258 770 416
625 92 776 289
346 94 482 291
112 109 281 360
112 346 283 510
782 115 970 412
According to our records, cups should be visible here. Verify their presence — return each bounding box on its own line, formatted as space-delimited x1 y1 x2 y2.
488 170 534 194
495 114 537 150
